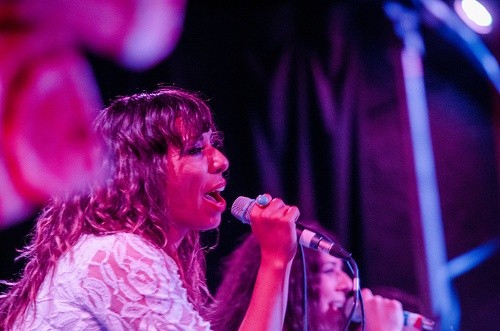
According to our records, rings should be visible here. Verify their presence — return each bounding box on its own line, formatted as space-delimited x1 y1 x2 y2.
254 194 270 208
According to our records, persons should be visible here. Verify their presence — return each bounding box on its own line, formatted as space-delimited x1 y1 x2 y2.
0 88 299 331
206 221 405 331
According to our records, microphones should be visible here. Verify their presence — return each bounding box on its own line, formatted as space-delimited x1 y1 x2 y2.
231 197 352 260
345 297 434 330
